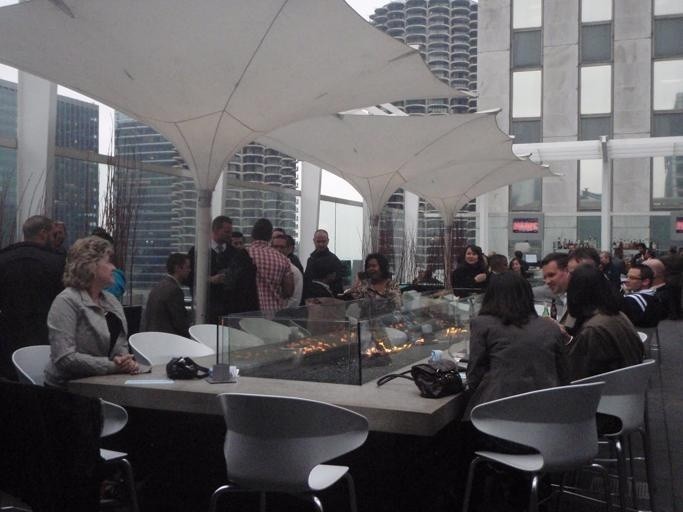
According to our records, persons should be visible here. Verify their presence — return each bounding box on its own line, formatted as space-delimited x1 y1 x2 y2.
452 242 526 296
52 220 64 245
91 226 127 301
44 236 140 454
0 214 66 379
543 239 682 437
139 216 399 335
465 270 558 510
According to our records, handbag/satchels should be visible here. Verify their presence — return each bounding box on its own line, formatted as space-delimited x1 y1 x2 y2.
282 272 294 298
411 359 465 398
167 357 199 379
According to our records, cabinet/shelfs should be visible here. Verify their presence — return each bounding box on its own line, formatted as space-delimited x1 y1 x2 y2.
612 247 653 267
553 249 600 258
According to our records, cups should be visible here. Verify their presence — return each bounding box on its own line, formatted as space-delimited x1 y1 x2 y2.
215 363 229 381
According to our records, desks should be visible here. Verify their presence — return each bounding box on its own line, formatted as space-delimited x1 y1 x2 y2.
63 288 574 512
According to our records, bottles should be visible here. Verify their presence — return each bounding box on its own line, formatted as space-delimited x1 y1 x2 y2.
612 238 656 249
541 299 557 320
551 237 589 249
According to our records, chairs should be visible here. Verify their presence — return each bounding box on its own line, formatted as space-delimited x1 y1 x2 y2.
127 330 221 370
181 323 266 357
635 324 660 344
9 334 140 511
648 281 665 303
455 377 617 512
565 351 662 512
633 330 650 350
208 388 376 511
241 317 312 347
